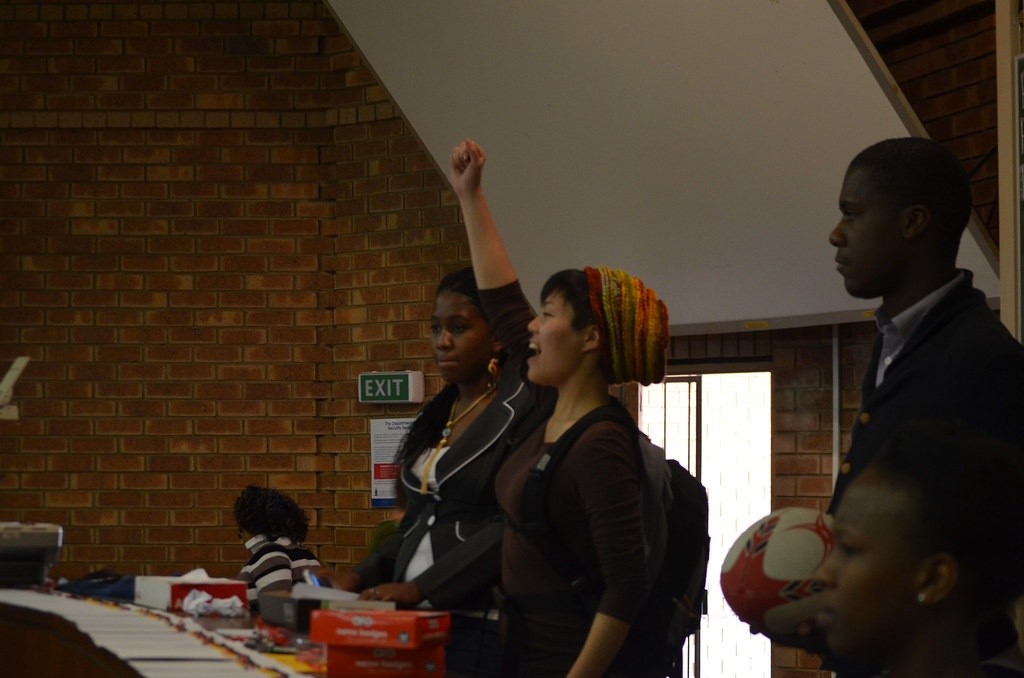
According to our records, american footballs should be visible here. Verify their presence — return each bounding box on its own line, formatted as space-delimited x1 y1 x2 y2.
722 506 839 647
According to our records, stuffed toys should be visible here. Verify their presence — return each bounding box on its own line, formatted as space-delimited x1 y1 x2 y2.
233 483 322 593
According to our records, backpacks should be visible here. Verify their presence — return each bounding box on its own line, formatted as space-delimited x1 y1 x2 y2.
526 401 712 678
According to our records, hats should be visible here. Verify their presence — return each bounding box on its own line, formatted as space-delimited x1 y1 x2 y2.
583 263 668 386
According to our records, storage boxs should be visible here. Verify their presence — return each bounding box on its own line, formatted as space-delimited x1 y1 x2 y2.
133 574 249 613
310 606 452 678
0 519 62 588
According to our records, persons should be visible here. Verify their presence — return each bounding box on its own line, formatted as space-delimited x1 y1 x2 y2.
822 136 1023 656
810 458 1024 678
440 141 676 678
313 263 555 677
